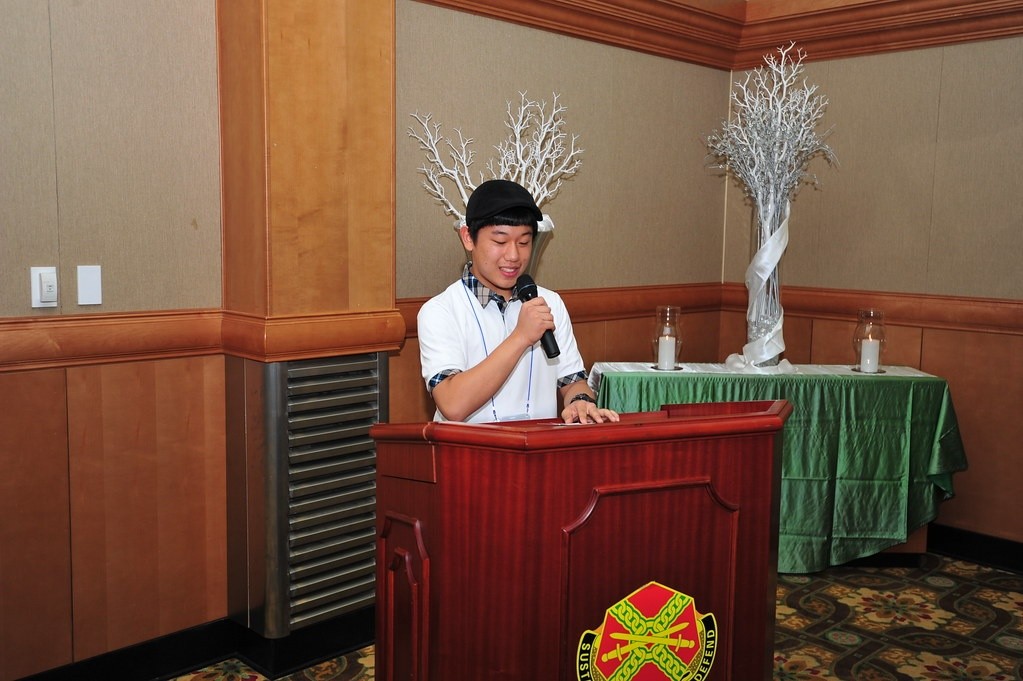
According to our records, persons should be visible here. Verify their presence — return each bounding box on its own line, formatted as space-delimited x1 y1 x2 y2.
415 180 620 426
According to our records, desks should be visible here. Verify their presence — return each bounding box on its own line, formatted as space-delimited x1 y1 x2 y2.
587 362 967 574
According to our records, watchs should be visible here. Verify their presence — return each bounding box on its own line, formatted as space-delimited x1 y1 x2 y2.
570 394 599 408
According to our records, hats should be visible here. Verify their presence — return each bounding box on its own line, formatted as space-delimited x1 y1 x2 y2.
466 180 543 226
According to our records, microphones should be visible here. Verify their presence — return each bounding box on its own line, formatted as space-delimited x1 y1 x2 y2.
516 274 560 359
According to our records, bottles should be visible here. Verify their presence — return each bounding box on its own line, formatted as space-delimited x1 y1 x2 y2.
852 308 887 372
652 305 683 371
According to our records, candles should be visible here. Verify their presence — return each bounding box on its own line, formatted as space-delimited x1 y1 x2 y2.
659 335 675 370
861 334 880 373
